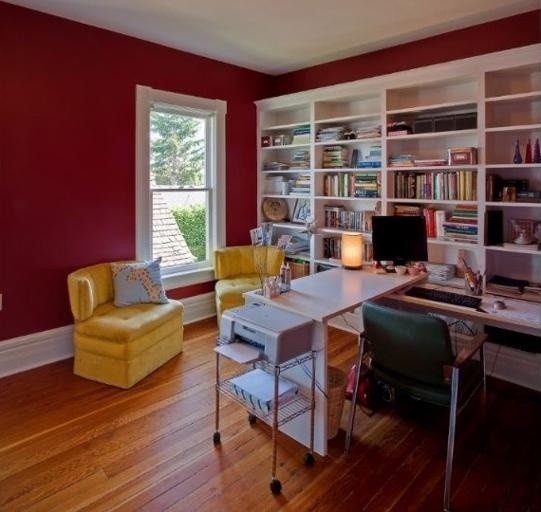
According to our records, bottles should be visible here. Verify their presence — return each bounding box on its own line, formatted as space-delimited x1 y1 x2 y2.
513 137 541 163
264 276 280 299
280 261 291 288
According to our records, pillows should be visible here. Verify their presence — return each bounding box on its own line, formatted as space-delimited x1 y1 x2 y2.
109 256 169 308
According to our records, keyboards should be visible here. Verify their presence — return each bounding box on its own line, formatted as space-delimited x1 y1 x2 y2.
405 286 482 309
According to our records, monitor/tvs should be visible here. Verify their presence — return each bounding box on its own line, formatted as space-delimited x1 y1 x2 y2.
372 215 428 273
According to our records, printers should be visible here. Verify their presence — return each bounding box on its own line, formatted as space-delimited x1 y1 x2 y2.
218 301 314 366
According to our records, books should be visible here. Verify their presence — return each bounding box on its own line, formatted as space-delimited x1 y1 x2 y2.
262 112 478 289
486 275 528 296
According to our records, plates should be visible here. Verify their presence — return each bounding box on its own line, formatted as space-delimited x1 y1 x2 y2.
262 198 288 220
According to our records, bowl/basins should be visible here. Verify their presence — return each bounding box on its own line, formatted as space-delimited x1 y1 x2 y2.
395 266 406 274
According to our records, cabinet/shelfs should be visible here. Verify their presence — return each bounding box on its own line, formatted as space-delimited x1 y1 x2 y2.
252 43 541 392
212 336 317 489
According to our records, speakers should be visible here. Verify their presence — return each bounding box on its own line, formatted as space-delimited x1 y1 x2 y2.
487 209 504 246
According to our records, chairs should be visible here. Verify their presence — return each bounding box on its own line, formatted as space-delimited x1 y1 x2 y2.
342 301 489 512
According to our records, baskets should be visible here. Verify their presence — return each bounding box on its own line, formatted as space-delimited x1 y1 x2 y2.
328 365 346 440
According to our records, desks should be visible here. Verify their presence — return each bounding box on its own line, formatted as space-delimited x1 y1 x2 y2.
242 264 430 457
368 280 541 338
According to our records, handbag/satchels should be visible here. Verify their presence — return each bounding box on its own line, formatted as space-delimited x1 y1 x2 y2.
346 362 379 413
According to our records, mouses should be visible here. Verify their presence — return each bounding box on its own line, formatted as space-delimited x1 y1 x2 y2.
493 301 506 310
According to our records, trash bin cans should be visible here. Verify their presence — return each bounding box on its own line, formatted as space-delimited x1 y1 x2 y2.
326 366 349 439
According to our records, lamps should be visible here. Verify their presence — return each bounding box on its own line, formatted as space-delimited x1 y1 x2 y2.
341 232 364 270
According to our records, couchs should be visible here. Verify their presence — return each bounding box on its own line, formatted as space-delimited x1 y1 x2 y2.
213 245 285 328
67 259 184 389
